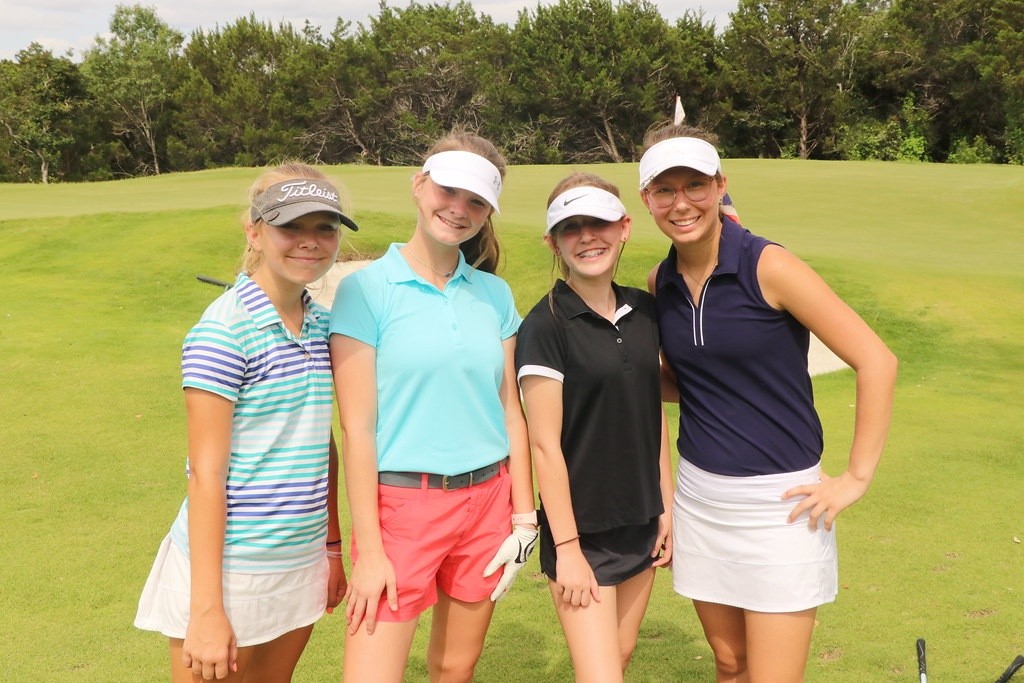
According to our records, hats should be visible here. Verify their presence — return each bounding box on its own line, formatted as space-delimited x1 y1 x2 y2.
546 187 627 234
249 179 358 232
422 152 503 215
638 137 725 193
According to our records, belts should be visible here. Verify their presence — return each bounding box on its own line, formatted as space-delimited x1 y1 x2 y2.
378 456 508 491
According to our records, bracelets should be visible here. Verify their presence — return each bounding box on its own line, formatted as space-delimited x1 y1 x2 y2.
326 539 341 547
554 535 580 547
327 551 342 558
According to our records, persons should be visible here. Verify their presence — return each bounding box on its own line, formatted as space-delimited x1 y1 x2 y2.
134 163 359 683
639 127 898 683
326 132 541 683
513 174 672 683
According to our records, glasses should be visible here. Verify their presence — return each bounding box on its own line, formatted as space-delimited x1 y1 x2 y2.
643 172 719 209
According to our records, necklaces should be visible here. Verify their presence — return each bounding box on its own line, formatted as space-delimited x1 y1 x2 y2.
405 244 458 277
680 262 703 287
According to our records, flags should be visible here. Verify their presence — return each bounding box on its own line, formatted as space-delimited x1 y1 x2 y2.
675 98 740 225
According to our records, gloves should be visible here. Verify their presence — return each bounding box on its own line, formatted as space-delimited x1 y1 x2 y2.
482 523 538 601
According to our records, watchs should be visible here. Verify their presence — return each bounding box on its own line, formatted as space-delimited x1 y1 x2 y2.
511 510 541 526
